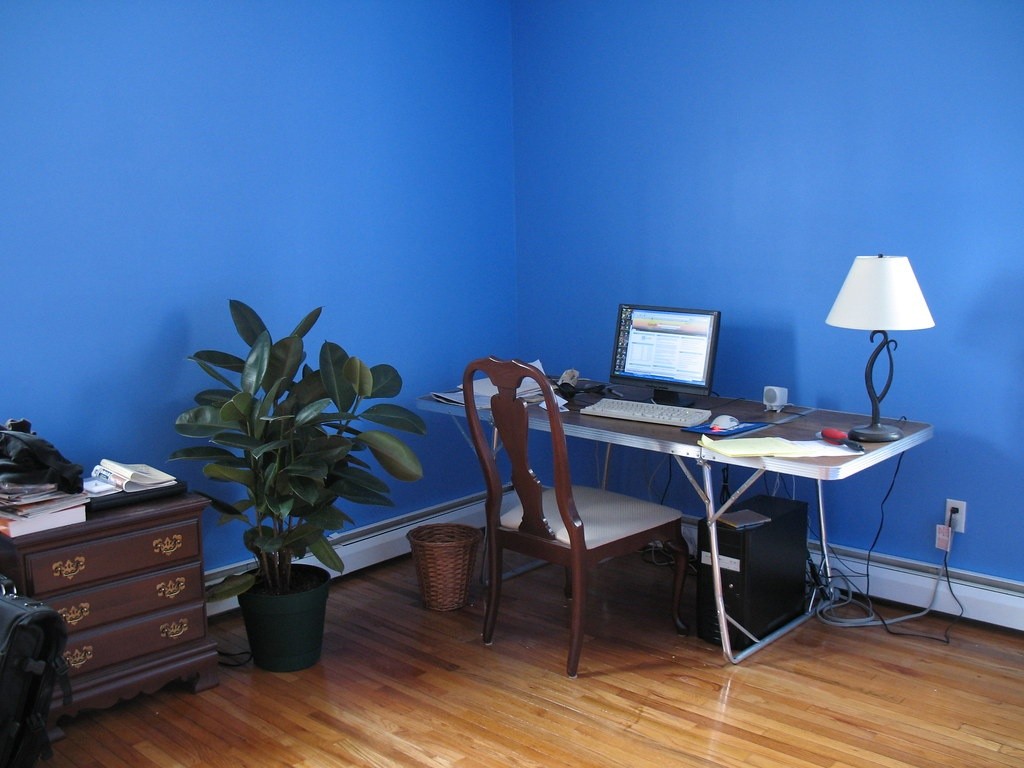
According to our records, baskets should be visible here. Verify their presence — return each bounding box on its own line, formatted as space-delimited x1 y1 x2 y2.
405 523 484 612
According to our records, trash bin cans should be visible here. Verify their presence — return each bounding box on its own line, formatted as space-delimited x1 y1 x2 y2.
405 523 478 611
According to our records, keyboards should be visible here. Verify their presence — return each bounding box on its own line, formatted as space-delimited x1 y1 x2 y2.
580 398 712 427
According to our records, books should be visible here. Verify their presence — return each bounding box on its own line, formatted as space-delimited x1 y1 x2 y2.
0 459 188 538
697 434 805 458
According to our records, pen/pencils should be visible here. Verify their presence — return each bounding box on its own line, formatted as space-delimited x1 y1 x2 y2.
606 387 624 398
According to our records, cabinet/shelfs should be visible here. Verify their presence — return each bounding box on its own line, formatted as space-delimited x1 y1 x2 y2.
0 490 219 744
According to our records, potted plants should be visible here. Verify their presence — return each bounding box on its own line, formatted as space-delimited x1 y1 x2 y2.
165 300 426 674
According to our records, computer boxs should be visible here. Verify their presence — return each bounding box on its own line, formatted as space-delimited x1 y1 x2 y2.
697 494 809 651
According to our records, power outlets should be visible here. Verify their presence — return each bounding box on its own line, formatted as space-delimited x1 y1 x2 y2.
945 499 966 533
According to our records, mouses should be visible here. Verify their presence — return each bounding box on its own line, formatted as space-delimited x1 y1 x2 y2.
710 415 740 430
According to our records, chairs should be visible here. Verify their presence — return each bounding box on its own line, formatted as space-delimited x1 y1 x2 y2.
462 356 690 678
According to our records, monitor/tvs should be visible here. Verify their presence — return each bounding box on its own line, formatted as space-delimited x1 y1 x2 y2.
609 304 721 408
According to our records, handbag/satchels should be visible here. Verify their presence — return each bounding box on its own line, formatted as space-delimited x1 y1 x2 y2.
0 575 73 768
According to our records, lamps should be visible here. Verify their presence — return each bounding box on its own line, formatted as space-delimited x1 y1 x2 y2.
825 252 935 442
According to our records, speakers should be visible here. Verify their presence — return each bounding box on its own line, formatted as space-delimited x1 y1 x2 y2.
763 386 788 412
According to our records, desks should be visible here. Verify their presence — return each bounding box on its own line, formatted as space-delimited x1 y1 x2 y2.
416 377 933 666
